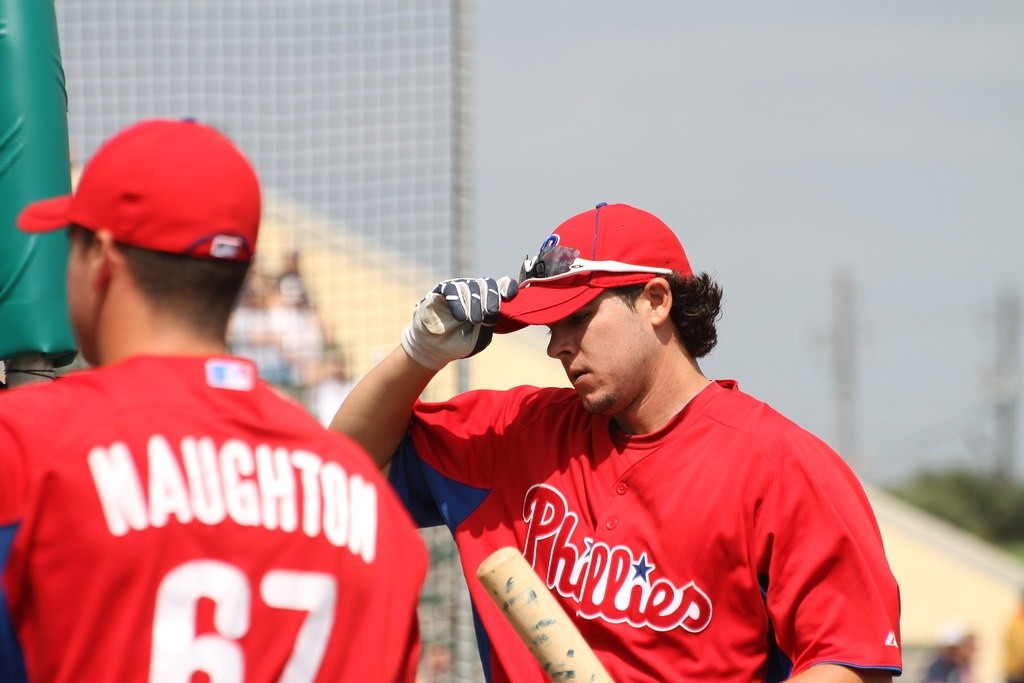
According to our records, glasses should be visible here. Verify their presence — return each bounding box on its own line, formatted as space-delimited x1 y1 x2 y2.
517 245 673 289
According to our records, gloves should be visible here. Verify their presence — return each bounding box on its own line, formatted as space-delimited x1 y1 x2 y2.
399 274 518 371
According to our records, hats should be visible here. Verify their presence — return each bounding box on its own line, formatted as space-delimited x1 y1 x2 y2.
493 203 693 334
15 116 261 260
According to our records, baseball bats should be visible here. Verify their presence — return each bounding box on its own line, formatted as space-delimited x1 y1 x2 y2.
477 546 613 682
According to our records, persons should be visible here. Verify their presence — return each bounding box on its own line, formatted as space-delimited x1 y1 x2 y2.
923 633 977 683
328 202 902 683
0 118 429 683
